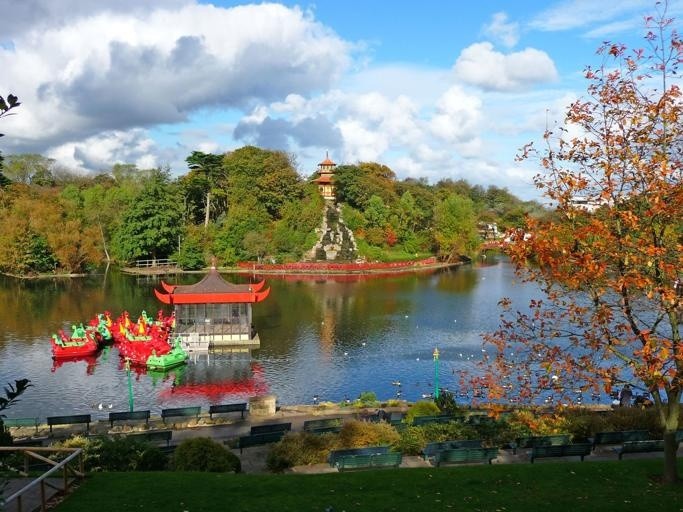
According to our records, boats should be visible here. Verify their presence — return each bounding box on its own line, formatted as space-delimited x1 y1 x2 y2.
47 305 187 372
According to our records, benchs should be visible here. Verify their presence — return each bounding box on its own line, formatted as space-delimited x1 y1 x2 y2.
47 414 92 432
109 410 150 428
237 421 291 456
208 402 247 420
131 433 177 456
161 406 201 424
